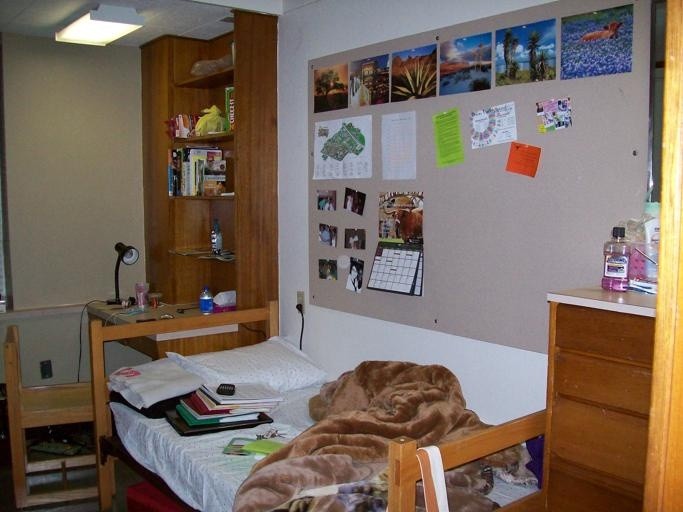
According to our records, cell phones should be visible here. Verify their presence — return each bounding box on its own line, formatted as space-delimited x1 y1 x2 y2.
217 383 235 396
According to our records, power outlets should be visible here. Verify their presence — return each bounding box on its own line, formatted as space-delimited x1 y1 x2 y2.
295 290 305 314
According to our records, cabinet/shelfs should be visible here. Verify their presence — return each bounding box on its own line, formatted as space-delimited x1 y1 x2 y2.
167 62 235 262
546 306 656 512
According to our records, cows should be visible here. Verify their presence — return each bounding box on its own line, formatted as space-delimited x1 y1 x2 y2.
382 203 423 244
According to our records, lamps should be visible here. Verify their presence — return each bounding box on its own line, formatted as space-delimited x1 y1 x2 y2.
108 241 140 304
52 3 144 47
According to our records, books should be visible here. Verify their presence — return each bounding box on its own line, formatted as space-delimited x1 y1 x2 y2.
165 145 227 202
174 114 201 139
224 87 235 132
163 379 285 436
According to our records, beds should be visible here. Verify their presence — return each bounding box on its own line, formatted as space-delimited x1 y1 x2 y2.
83 298 543 512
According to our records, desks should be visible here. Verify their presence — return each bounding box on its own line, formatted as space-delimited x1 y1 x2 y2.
85 299 239 363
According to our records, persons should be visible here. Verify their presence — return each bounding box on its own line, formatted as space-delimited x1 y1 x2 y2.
321 264 337 280
345 261 361 293
319 224 336 247
346 192 354 211
318 196 335 211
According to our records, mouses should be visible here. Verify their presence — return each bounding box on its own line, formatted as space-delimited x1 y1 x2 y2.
161 313 174 319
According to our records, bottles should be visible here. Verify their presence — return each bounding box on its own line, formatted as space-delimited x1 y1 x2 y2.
199 287 214 315
601 226 631 293
